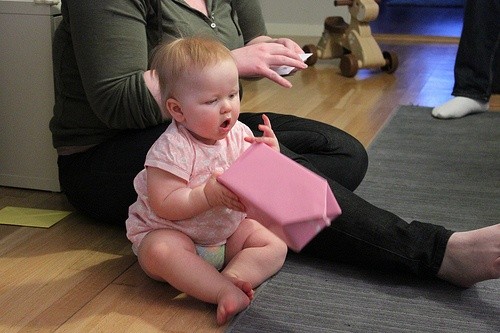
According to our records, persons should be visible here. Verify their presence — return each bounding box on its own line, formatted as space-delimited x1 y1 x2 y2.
125 33 288 326
432 0 500 118
49 0 500 287
